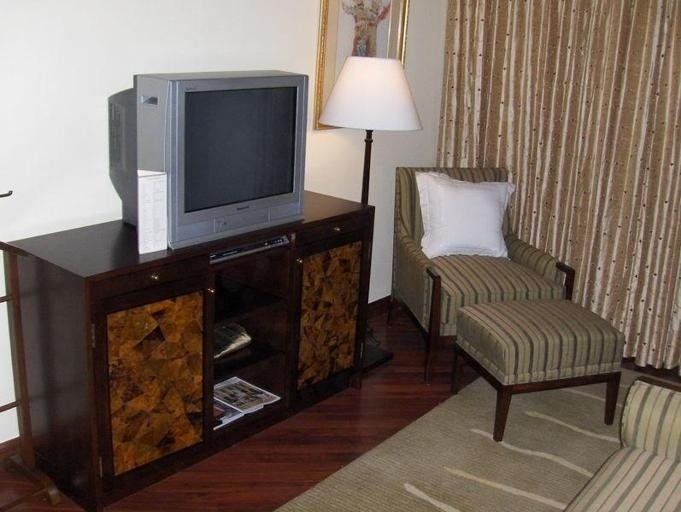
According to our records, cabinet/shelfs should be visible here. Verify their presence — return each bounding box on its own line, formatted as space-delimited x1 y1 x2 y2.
2 192 375 510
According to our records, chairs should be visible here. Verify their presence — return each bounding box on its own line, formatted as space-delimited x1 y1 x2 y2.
386 167 576 385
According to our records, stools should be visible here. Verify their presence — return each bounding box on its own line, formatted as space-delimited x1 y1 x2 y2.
452 300 625 442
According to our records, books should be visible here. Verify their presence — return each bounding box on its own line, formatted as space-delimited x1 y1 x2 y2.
213 376 282 430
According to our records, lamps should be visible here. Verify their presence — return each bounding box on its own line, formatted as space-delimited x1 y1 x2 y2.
320 57 424 375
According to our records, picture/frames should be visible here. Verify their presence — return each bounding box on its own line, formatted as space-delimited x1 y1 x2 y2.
314 1 411 133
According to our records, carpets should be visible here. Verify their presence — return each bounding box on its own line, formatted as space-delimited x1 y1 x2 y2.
276 368 681 512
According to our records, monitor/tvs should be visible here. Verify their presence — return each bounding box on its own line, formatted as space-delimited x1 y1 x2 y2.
108 69 309 256
